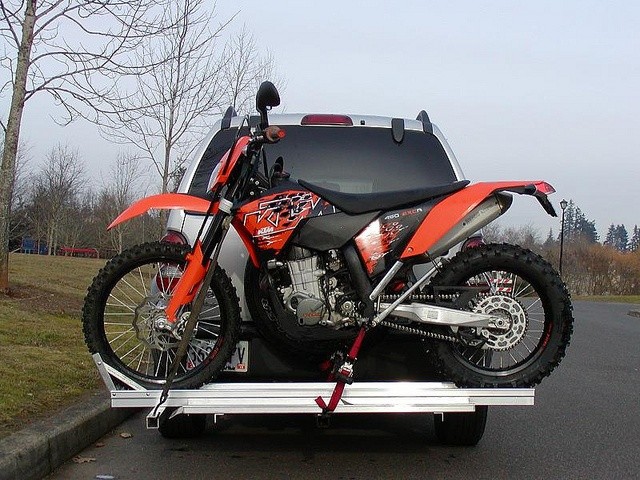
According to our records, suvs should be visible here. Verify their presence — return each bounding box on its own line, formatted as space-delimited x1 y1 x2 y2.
150 107 489 446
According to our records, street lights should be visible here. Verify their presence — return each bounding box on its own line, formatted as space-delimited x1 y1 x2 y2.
559 198 568 275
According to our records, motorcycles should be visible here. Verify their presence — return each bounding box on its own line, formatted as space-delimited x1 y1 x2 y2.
82 81 574 389
